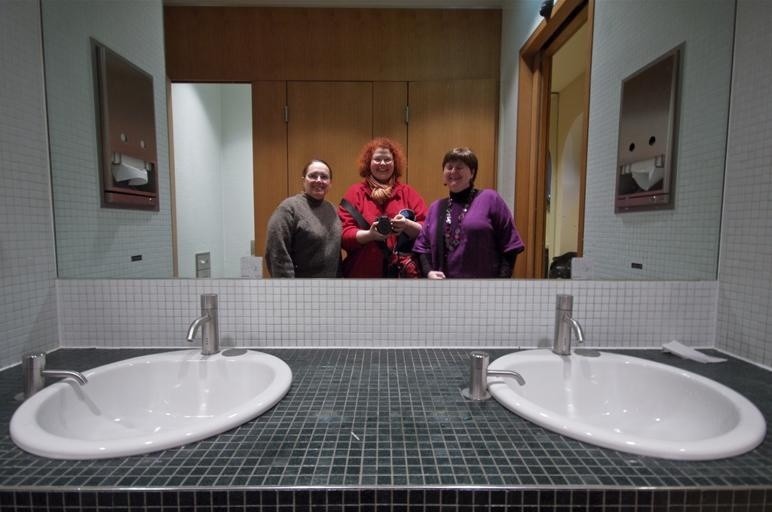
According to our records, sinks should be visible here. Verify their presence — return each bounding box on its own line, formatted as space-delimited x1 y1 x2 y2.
9 349 294 459
486 348 768 463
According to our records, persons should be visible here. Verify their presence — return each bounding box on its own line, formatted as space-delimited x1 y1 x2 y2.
265 158 343 278
410 145 525 277
337 136 427 278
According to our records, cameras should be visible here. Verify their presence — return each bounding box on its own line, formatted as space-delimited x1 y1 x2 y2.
376 216 397 234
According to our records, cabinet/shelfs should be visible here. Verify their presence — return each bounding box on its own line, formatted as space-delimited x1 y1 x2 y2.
374 77 494 258
251 81 373 257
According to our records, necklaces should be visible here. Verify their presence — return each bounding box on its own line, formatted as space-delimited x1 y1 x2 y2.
445 188 475 254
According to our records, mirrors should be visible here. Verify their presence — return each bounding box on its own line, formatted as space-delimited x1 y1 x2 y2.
39 0 740 282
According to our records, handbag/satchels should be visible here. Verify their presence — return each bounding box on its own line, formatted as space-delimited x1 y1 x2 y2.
382 248 423 278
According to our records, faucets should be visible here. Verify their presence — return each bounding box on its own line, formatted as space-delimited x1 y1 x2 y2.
552 293 585 357
468 349 525 399
185 293 220 357
23 350 88 401
